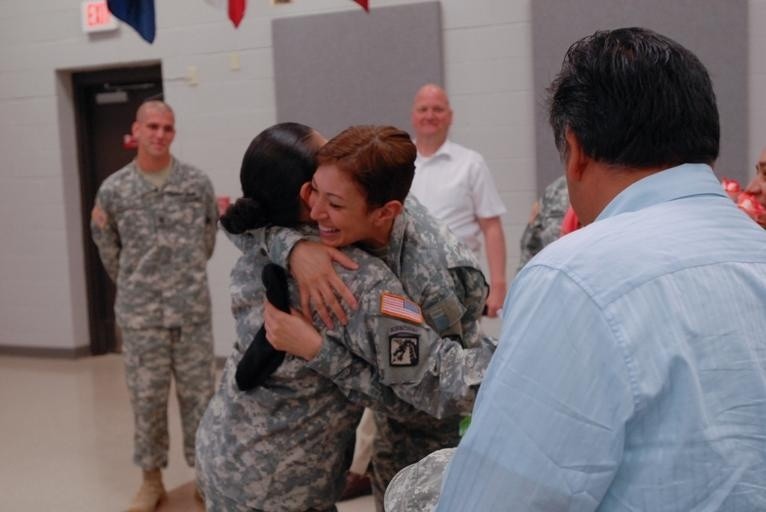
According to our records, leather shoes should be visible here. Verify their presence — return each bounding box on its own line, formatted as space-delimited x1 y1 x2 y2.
339 470 373 501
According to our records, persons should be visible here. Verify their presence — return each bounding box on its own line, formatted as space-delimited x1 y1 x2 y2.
403 83 507 318
435 27 766 512
513 174 586 276
89 102 221 512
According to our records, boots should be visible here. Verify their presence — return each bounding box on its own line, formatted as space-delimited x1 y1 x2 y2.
128 467 165 512
